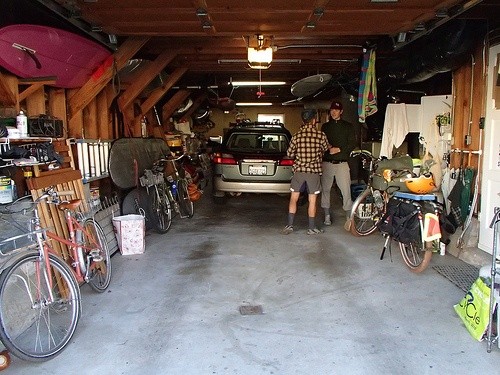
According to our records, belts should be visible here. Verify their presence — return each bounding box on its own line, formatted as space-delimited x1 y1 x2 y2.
322 160 348 164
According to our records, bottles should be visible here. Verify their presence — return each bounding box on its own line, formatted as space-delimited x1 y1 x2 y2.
24 171 33 195
440 241 445 255
16 110 27 137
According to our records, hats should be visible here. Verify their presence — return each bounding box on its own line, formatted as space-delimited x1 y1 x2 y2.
330 101 343 109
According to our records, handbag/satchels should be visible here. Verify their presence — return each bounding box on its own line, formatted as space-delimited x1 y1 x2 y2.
454 276 497 342
376 198 420 248
436 201 456 246
112 208 145 255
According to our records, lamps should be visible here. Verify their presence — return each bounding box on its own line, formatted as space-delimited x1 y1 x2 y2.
246 36 273 70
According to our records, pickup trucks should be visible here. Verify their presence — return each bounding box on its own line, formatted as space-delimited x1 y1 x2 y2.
212 120 296 206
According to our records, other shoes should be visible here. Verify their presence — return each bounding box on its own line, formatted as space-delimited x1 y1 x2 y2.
280 225 293 234
346 213 350 220
324 215 331 226
306 228 324 235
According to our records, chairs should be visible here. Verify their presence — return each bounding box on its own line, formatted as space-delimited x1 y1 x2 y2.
237 138 251 148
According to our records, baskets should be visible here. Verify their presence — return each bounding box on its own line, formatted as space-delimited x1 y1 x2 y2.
0 198 38 252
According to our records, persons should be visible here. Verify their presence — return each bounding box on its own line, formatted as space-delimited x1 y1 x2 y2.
320 101 357 225
280 109 332 235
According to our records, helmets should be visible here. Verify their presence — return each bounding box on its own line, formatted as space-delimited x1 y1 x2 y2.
406 174 435 194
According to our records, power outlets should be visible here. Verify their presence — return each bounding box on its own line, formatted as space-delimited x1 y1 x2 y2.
465 135 471 145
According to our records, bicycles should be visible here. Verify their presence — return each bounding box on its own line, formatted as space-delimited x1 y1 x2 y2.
350 148 438 272
140 152 194 233
0 178 112 362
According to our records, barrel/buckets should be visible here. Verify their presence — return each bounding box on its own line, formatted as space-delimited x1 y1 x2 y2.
0 176 13 204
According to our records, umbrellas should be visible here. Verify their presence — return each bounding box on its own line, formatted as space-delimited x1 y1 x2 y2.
441 149 474 228
